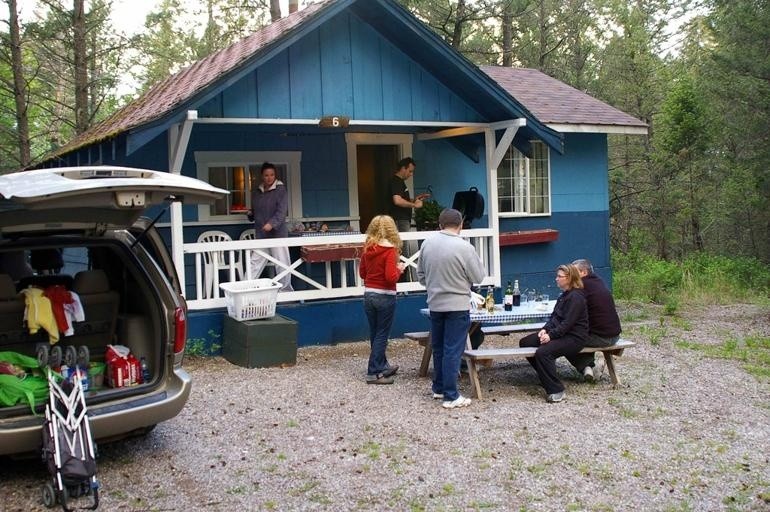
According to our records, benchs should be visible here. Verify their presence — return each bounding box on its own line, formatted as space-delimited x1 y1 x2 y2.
464 340 635 399
404 322 546 376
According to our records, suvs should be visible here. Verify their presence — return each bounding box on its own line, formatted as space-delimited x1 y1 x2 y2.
0 165 233 458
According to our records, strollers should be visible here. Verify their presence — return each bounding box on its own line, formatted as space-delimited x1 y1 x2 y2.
38 346 103 512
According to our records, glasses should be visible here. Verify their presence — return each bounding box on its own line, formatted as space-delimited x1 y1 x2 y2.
555 274 570 279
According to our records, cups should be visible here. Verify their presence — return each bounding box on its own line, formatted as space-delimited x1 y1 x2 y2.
520 291 550 311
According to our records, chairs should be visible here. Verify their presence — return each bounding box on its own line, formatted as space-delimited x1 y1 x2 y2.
0 274 35 357
62 270 118 361
433 187 484 231
239 228 274 280
198 230 242 298
17 249 73 291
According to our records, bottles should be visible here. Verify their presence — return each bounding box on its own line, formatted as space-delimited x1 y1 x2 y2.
485 285 496 315
138 356 152 385
503 280 514 312
512 279 521 306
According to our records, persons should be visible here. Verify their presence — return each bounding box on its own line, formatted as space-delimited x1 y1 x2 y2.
519 264 590 402
459 281 484 374
416 207 487 409
242 161 295 293
380 156 424 282
358 214 405 384
563 258 622 384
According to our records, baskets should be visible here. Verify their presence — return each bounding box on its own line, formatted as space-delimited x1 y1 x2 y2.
218 277 284 324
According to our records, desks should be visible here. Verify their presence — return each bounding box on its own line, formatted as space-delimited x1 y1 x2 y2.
300 244 364 286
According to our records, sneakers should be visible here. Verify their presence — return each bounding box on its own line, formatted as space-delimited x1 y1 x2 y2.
365 373 394 386
381 363 399 377
432 390 445 401
592 356 607 383
441 393 474 410
582 365 595 384
461 364 486 374
545 389 568 403
